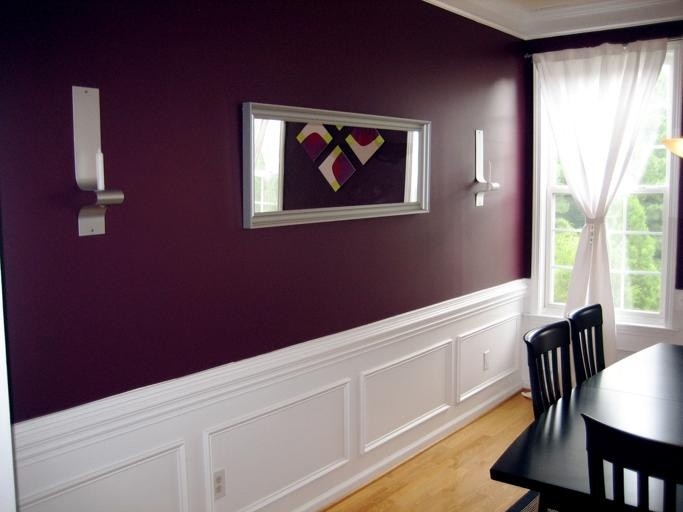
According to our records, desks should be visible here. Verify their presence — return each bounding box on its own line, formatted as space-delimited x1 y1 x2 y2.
488 342 683 512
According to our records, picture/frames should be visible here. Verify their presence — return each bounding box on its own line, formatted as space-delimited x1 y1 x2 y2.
242 101 432 229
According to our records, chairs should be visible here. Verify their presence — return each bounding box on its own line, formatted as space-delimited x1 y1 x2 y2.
566 303 606 386
523 319 571 421
581 412 683 512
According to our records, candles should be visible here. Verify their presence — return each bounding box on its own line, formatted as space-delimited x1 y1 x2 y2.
488 158 492 181
95 149 105 191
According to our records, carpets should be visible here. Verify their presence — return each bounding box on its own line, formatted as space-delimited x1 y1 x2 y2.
505 489 560 512
